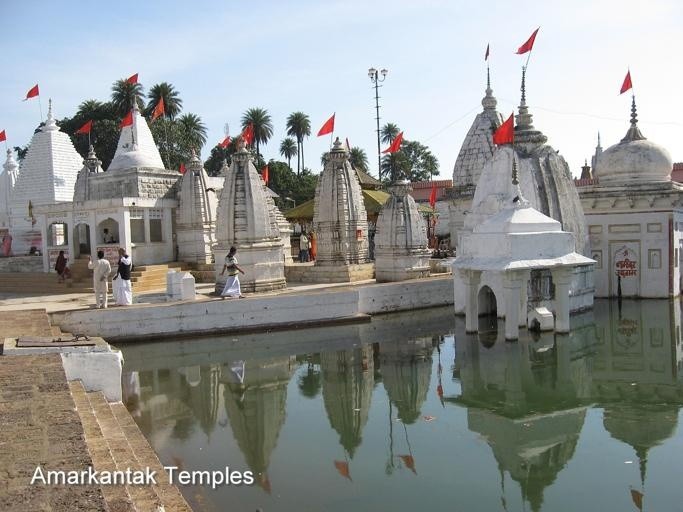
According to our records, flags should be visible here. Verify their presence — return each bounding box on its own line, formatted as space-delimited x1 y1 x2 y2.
74 73 268 185
436 384 444 398
428 184 435 206
171 459 184 472
515 28 537 55
630 487 644 511
333 459 352 482
381 132 402 154
259 479 270 492
0 129 5 142
397 454 417 475
483 45 489 61
26 84 39 98
492 114 512 145
619 72 632 94
316 115 333 137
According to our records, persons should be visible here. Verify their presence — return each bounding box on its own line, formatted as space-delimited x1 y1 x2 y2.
87 251 110 308
171 233 179 261
55 251 71 283
121 370 142 420
111 247 132 306
218 246 246 300
223 360 251 409
102 228 115 243
298 230 317 263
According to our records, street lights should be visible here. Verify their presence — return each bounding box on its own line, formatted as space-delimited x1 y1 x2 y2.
367 67 388 184
